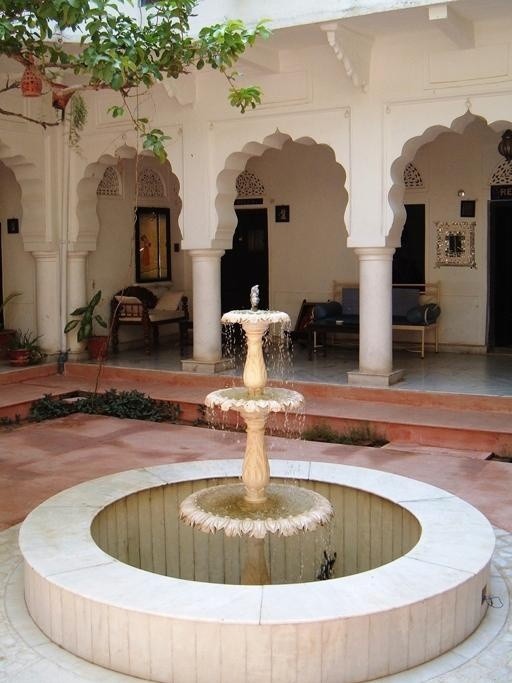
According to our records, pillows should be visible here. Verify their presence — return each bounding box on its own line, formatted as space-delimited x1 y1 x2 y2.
114 295 150 314
154 291 185 312
407 303 441 323
314 301 342 320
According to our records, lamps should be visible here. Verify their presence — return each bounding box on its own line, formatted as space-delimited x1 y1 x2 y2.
498 129 512 162
275 205 289 222
21 0 42 97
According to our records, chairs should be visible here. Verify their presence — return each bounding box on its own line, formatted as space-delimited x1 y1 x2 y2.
111 286 188 353
286 300 331 358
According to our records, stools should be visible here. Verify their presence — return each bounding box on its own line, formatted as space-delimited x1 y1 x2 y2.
179 320 193 356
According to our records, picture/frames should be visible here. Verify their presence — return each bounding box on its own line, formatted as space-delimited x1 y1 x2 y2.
134 207 171 282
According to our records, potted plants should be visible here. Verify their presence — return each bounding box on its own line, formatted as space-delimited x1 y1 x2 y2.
63 289 110 362
0 289 48 367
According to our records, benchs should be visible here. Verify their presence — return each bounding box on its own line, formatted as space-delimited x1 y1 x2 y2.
312 280 441 359
